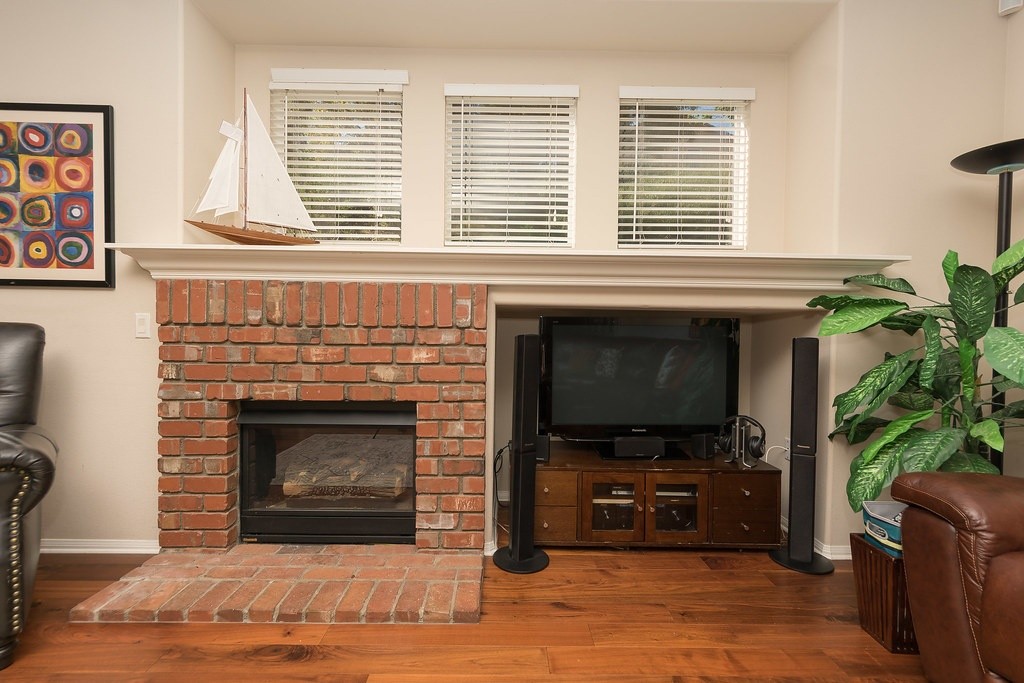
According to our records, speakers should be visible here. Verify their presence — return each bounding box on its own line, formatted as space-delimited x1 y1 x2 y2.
493 333 549 574
769 336 834 574
613 437 665 458
691 434 716 460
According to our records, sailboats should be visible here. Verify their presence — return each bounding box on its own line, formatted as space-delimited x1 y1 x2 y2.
182 86 322 246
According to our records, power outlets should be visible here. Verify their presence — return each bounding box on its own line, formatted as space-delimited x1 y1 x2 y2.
785 438 790 460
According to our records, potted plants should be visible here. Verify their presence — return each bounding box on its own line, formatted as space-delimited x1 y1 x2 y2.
807 240 1024 653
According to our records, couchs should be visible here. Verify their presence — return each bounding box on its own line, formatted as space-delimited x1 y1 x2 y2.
892 472 1024 683
0 322 59 671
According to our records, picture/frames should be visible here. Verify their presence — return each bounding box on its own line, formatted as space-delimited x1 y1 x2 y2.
0 102 116 290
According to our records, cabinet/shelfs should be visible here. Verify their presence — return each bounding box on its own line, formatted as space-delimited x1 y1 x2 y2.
508 441 782 552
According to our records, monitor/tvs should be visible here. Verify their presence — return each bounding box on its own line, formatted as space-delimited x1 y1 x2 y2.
537 316 741 461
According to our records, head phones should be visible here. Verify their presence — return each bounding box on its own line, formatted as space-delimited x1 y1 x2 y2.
717 415 766 459
